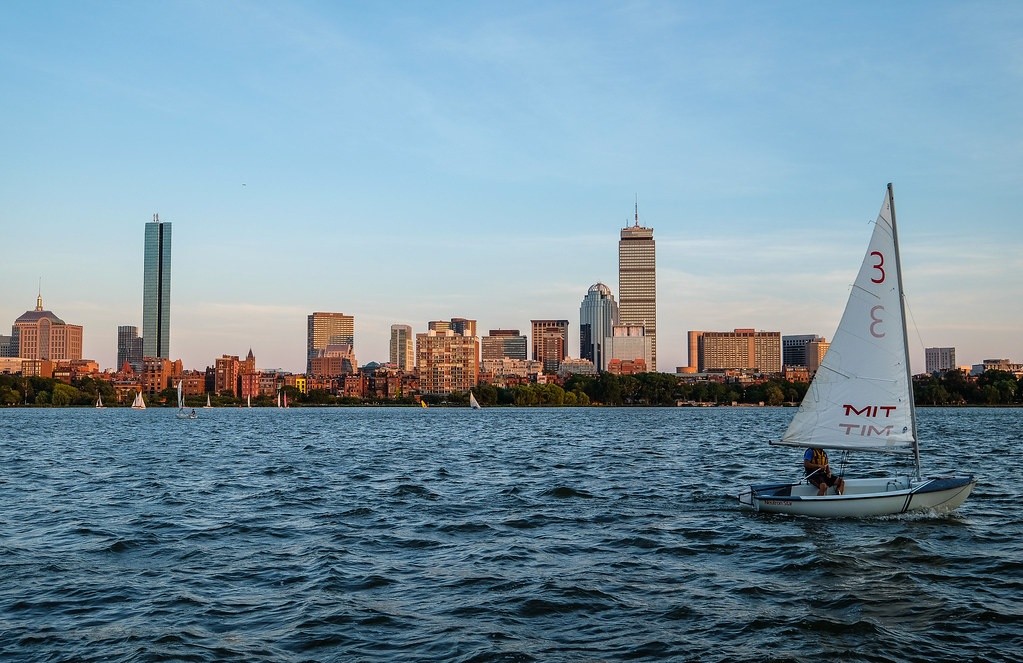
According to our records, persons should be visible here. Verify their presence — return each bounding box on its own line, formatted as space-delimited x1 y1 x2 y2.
803 447 844 496
192 409 195 415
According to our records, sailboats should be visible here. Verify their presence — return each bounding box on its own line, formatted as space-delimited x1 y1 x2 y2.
174 379 198 419
247 394 252 409
277 391 282 409
283 390 290 409
203 392 214 408
95 393 107 409
737 182 979 517
131 389 146 410
421 399 427 408
469 391 481 410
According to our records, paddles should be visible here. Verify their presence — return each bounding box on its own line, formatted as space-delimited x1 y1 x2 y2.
800 461 831 482
